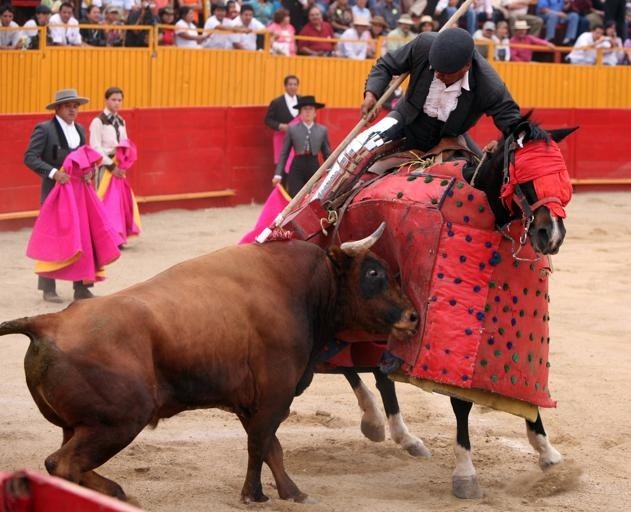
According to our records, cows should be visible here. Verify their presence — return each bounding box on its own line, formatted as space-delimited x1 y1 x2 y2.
0 222 420 504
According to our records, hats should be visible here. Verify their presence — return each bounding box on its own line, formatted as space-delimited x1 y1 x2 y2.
211 0 228 16
293 96 324 109
47 90 88 109
106 4 120 14
429 28 474 74
36 6 50 14
350 14 531 29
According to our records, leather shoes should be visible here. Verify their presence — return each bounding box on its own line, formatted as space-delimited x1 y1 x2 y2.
44 291 63 303
74 288 95 300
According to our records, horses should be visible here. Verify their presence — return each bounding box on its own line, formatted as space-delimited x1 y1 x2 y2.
344 110 580 498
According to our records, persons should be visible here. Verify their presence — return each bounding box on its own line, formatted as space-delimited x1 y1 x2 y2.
1 0 338 66
328 0 631 66
91 85 133 254
270 95 337 199
360 26 525 160
262 75 306 173
24 90 99 303
